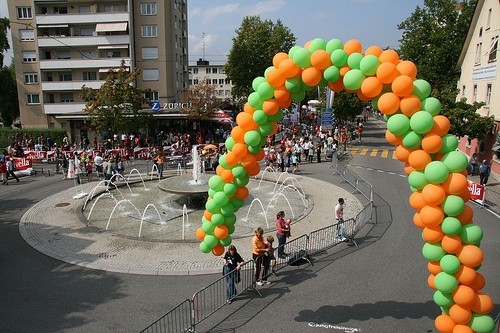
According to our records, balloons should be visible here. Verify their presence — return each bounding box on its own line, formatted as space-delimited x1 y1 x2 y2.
196 35 497 333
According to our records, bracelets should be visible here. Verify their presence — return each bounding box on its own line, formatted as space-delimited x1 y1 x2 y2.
239 263 242 267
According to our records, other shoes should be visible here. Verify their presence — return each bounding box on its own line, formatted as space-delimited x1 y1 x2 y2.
279 255 285 259
262 280 272 284
256 281 264 286
284 252 288 256
227 299 231 303
338 237 346 242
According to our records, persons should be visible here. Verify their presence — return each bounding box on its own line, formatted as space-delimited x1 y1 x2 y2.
253 227 275 285
262 235 277 285
223 245 245 303
282 217 292 237
479 159 491 187
0 100 385 186
470 153 479 176
276 210 289 259
335 198 347 242
